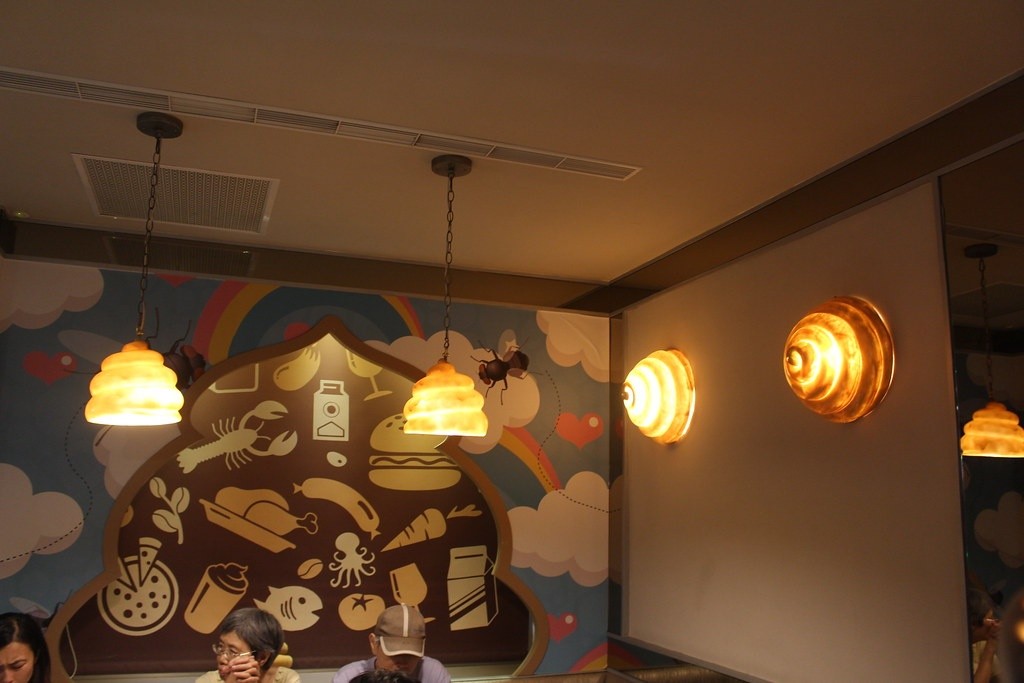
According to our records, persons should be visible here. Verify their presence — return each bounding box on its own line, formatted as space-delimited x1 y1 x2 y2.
334 604 452 683
0 611 53 683
967 586 1007 683
195 607 299 683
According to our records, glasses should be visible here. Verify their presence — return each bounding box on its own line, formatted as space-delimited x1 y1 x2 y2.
984 618 1001 626
212 644 256 658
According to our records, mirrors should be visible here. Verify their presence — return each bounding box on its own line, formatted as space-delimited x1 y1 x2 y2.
932 132 1024 682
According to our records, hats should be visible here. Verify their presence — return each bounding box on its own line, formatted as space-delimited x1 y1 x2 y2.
375 603 427 658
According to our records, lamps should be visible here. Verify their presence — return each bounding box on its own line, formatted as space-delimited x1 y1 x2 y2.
961 243 1023 457
620 349 695 444
84 110 183 427
401 155 488 437
784 296 895 425
